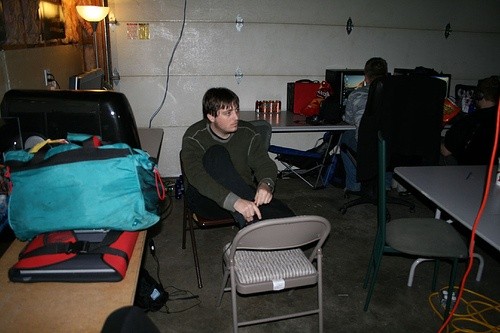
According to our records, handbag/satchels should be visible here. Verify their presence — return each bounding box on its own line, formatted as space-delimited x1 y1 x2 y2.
3 131 167 242
303 81 333 118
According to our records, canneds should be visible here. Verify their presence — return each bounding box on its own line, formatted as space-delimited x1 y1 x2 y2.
274 100 281 113
255 112 280 126
268 101 274 113
262 100 268 113
255 100 262 113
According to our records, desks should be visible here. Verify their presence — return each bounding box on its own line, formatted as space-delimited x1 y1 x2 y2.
0 230 147 333
236 110 356 190
138 126 164 163
392 164 500 254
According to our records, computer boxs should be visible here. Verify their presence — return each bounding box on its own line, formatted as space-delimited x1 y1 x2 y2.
324 68 366 121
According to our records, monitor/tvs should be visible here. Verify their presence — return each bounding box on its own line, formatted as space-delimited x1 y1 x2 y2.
339 72 366 106
432 73 451 98
69 67 104 91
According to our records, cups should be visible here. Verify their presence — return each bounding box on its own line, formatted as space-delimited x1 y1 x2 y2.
496 158 500 186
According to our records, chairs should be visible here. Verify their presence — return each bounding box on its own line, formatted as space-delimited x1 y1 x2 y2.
214 215 332 333
180 149 236 289
334 77 448 221
267 132 343 186
361 139 467 316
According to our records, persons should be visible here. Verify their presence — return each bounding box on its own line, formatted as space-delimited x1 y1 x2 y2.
440 75 500 166
182 87 319 251
339 58 393 198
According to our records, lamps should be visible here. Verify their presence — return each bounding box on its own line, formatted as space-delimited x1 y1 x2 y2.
75 5 109 34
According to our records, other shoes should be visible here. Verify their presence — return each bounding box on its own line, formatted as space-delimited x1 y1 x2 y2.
344 190 361 200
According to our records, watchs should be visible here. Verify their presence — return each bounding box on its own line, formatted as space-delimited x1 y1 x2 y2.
258 179 274 193
